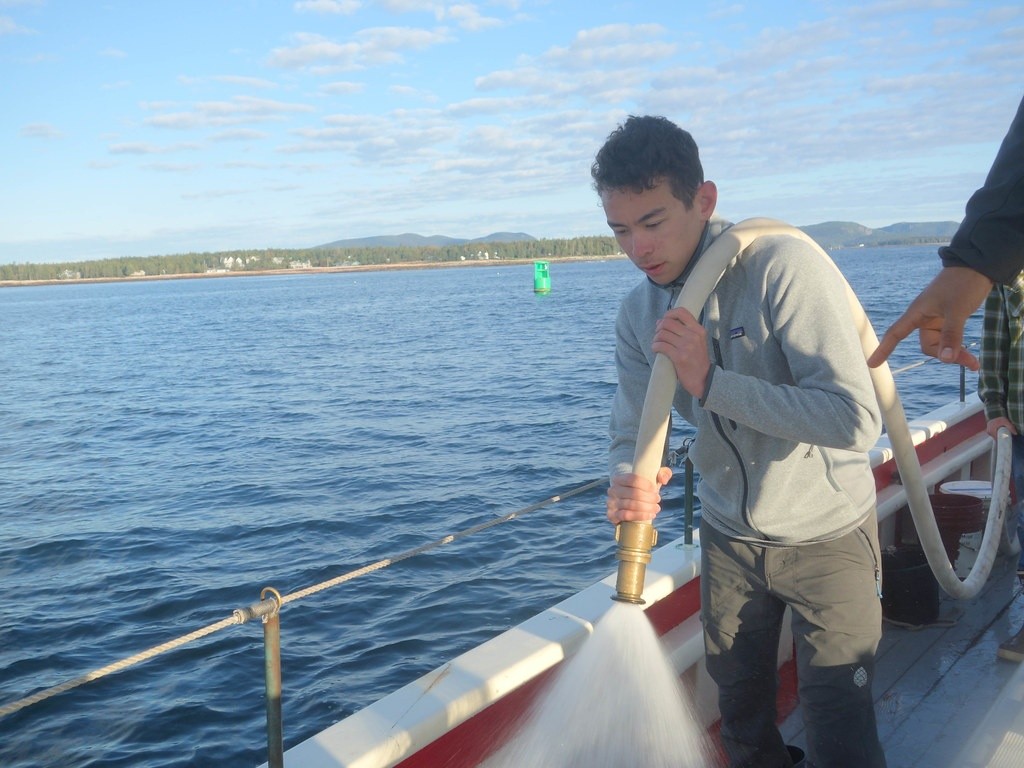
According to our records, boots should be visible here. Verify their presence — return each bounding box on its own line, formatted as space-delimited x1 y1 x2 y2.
997 571 1024 661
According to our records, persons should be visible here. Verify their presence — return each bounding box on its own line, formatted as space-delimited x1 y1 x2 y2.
866 93 1024 368
977 268 1024 663
589 116 887 768
